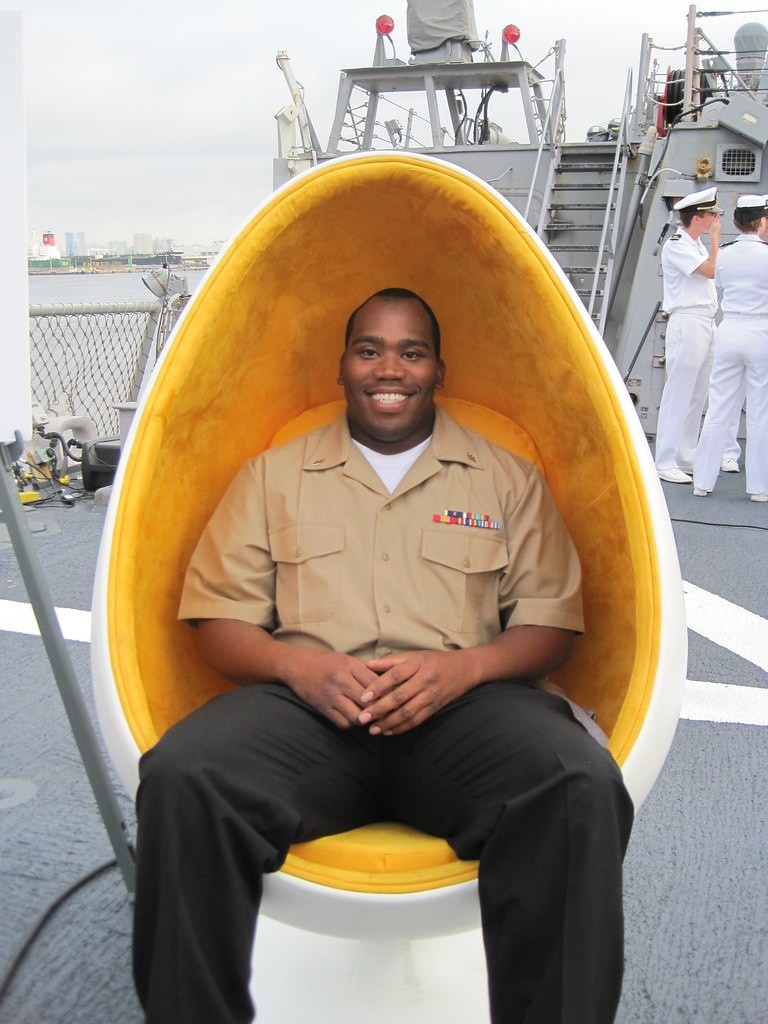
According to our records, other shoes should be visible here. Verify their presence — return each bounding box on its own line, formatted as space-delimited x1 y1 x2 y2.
750 491 768 502
693 486 713 496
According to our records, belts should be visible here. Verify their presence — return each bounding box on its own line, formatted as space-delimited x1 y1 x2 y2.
672 307 719 318
723 313 768 319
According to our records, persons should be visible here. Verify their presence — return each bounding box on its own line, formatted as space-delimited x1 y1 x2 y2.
654 187 724 484
691 194 768 504
131 286 636 1024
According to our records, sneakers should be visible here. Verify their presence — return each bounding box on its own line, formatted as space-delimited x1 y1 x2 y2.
678 466 693 474
721 458 740 473
656 467 693 483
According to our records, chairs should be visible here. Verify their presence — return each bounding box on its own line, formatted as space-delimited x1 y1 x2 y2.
93 152 687 1024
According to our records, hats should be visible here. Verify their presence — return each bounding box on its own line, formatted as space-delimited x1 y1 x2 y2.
737 194 768 210
674 187 724 213
